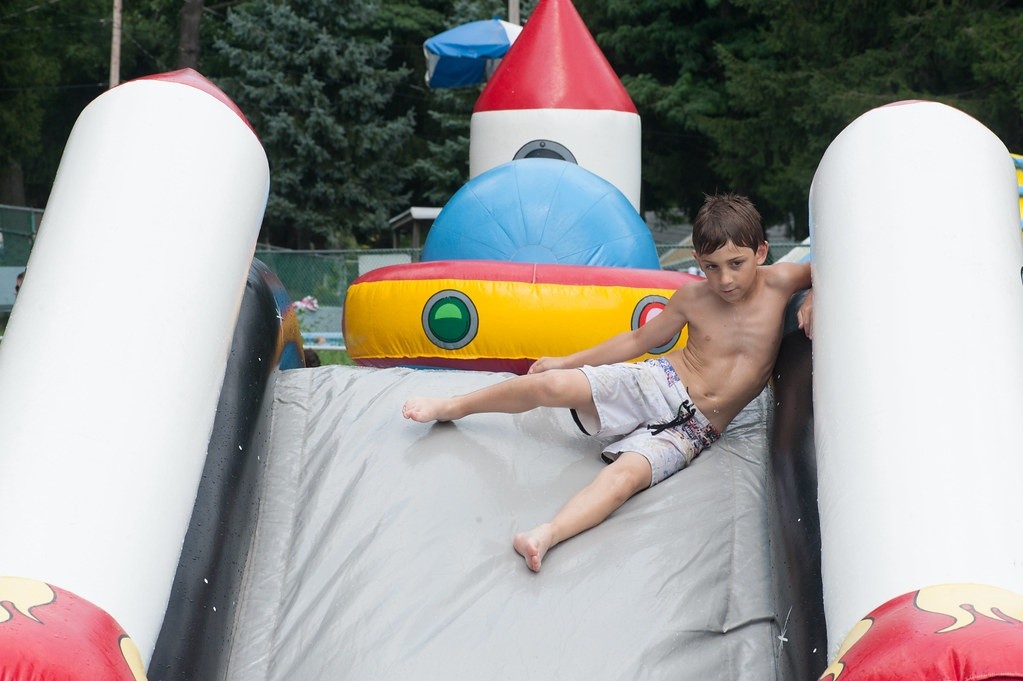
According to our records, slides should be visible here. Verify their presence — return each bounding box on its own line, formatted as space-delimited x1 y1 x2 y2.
196 359 787 680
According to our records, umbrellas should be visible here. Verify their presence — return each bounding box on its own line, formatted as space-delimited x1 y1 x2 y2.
424 15 523 94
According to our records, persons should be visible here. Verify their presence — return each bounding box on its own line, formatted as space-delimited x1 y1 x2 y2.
303 349 324 368
401 195 815 573
13 273 24 296
